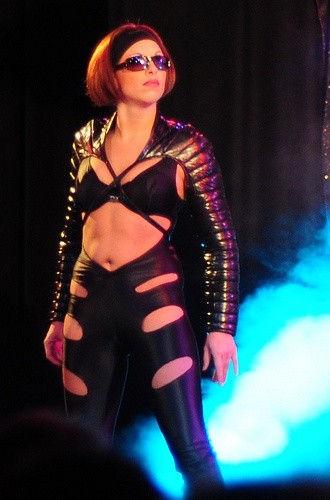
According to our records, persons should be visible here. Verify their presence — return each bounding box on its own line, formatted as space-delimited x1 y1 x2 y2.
43 20 233 500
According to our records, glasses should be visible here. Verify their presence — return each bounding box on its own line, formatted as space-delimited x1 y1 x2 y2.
113 55 171 72
